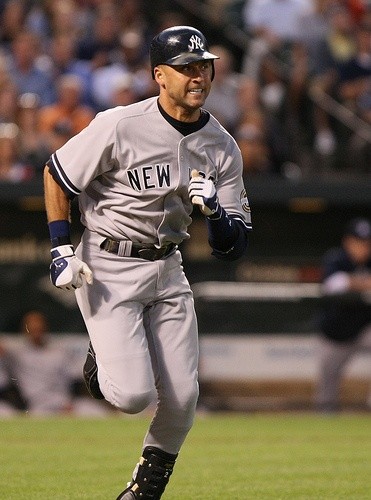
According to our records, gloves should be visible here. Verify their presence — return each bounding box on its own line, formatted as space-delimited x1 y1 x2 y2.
186 167 222 217
46 243 95 293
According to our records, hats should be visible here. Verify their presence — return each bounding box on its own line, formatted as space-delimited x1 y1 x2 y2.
148 24 221 69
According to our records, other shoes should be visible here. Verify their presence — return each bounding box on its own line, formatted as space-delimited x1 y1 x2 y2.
114 443 178 500
81 340 106 401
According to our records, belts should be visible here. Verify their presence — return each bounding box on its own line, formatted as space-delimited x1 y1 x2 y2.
100 236 178 262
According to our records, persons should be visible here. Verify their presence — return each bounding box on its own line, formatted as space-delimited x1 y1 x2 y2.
0 0 371 415
44 26 252 500
